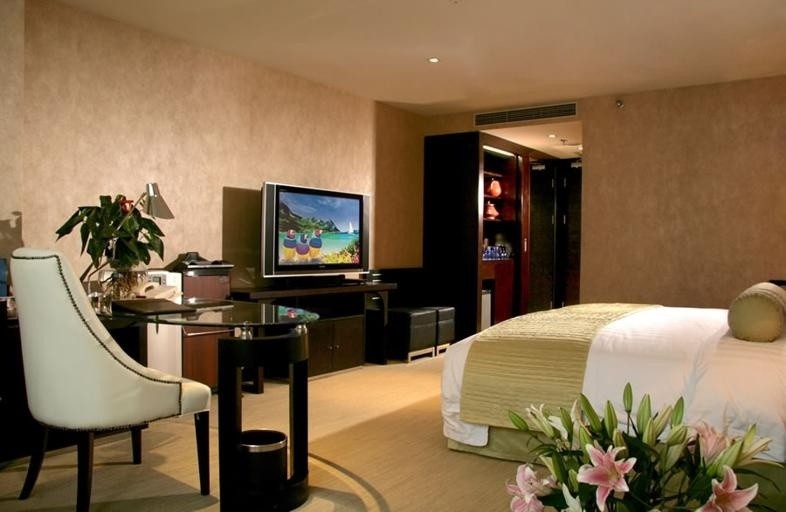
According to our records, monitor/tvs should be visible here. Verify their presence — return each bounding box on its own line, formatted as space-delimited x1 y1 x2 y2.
259 181 372 283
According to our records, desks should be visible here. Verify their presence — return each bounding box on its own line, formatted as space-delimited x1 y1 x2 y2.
95 295 320 512
231 280 397 394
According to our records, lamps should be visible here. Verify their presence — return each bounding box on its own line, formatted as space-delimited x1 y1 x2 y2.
87 182 175 310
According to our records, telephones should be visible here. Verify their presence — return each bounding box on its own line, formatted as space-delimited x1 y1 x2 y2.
134 281 179 302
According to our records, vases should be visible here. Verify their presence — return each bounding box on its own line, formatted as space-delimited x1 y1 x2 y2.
98 258 149 301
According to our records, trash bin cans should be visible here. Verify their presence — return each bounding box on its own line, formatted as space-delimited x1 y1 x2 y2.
241 429 288 488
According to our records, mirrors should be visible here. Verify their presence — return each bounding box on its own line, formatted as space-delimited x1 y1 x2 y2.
527 155 556 313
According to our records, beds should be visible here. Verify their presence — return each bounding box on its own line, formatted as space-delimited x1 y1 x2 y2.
440 303 786 465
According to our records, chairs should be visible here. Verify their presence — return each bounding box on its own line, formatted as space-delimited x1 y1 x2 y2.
9 248 212 512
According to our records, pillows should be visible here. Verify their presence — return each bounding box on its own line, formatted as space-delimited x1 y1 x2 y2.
728 281 786 343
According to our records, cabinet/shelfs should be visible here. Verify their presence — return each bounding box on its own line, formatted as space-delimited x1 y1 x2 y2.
182 271 230 393
0 305 148 464
422 130 527 333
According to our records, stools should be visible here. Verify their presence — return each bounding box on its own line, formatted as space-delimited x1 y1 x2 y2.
388 306 455 363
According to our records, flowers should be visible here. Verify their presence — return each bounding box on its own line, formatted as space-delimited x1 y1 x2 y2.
503 382 786 512
55 194 165 286
